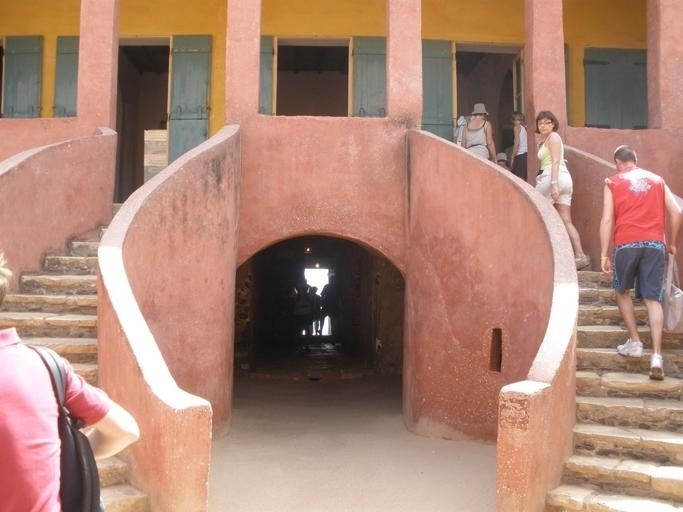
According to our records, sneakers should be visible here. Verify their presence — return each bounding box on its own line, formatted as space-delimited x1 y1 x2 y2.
649 356 664 380
576 256 589 270
617 337 643 358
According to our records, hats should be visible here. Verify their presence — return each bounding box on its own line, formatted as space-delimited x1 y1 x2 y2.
497 153 508 161
472 104 488 114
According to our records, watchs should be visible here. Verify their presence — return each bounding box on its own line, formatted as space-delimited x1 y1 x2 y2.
552 180 558 184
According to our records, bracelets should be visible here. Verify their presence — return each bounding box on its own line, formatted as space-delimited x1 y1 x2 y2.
599 256 609 260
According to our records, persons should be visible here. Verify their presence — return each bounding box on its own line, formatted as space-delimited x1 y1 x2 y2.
0 252 140 512
291 273 340 346
534 110 589 271
600 143 682 381
456 103 527 184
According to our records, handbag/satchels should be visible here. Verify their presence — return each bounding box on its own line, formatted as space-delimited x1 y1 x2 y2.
662 279 683 334
58 407 105 512
